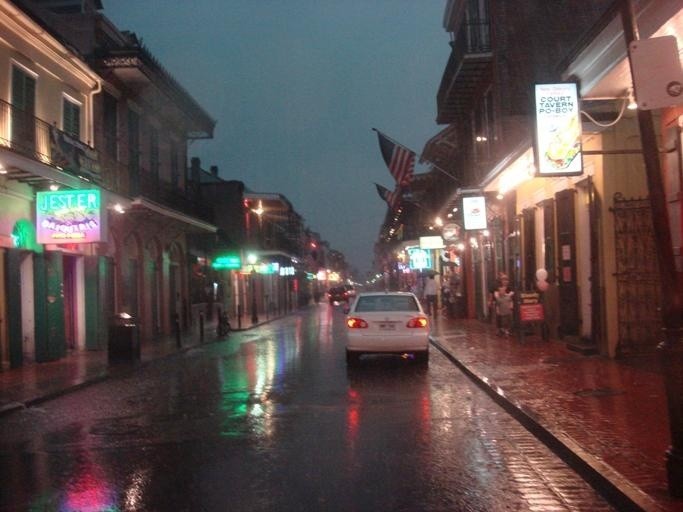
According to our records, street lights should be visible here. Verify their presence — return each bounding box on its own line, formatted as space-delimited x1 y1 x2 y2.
246 254 259 324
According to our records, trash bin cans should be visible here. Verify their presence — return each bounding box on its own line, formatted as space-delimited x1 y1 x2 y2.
108 312 138 363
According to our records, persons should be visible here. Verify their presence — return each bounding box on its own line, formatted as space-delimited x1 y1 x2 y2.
423 266 465 320
487 272 516 338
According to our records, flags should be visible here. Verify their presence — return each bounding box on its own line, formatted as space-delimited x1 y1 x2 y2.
377 184 402 215
378 133 418 191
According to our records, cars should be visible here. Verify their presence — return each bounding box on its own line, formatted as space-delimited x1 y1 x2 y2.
327 284 431 368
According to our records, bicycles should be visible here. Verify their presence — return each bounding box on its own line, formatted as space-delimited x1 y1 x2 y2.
216 310 231 337
